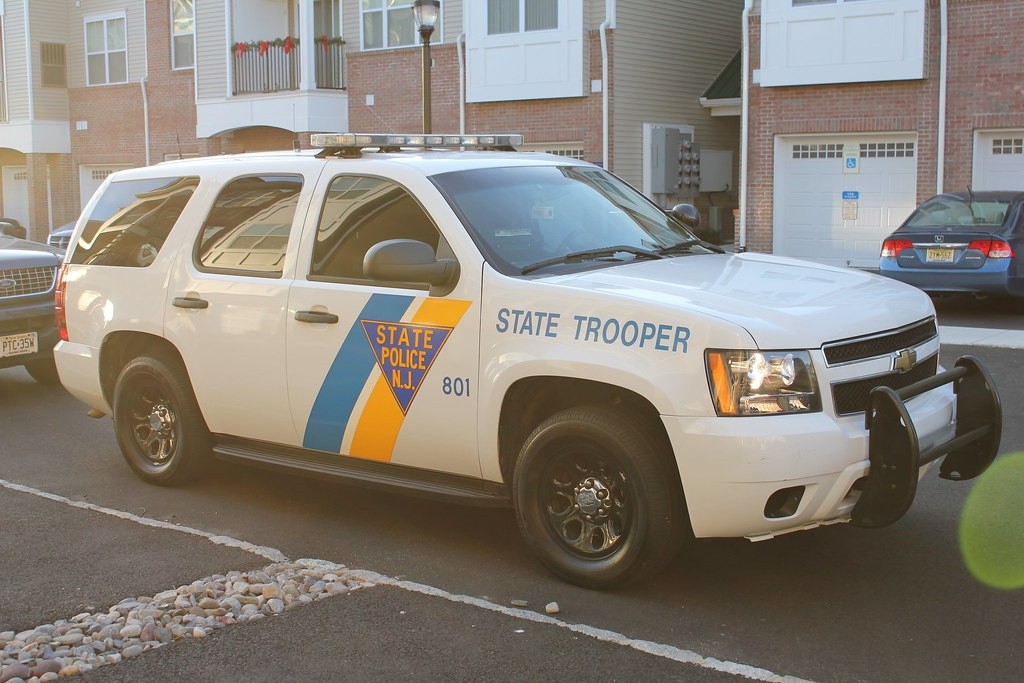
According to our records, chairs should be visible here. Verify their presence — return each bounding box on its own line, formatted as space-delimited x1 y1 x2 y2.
478 218 544 258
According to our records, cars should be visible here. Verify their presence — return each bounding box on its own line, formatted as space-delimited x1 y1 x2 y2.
878 184 1024 308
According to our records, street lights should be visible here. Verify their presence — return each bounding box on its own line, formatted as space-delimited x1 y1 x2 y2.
412 0 441 134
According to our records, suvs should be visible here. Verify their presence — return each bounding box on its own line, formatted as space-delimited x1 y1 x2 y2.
54 133 1004 589
0 221 67 385
